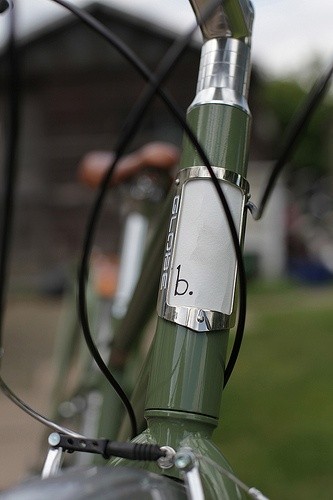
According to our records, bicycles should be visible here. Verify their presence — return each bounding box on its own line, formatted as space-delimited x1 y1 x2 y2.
0 1 331 500
